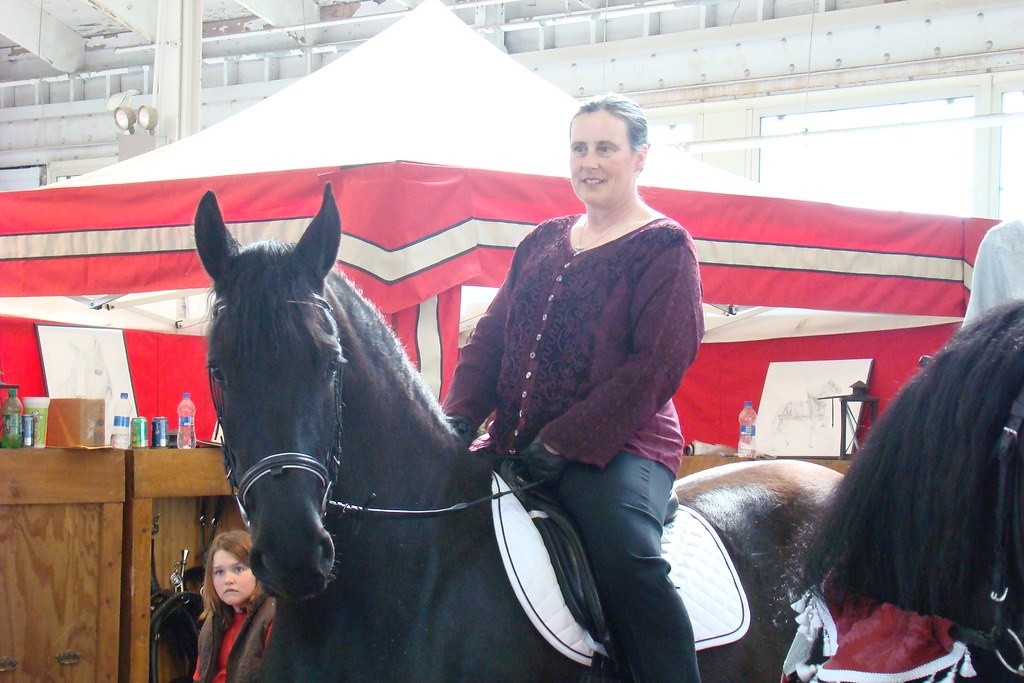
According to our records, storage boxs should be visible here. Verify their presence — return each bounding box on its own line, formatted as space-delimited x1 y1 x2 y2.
45 398 106 447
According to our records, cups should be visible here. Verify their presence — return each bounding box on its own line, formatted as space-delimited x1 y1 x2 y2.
23 396 50 449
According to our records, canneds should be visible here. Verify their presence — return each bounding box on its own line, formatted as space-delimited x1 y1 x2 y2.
131 417 148 448
21 415 35 447
152 417 169 448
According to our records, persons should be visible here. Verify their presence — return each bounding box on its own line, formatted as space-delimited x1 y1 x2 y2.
442 93 704 683
194 530 276 683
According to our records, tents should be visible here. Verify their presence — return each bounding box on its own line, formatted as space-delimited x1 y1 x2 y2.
0 0 1002 455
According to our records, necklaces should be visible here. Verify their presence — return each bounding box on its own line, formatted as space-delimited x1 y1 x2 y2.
576 217 626 250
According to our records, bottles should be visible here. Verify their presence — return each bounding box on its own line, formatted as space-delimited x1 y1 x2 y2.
110 392 132 449
2 389 23 449
176 392 197 449
737 400 758 459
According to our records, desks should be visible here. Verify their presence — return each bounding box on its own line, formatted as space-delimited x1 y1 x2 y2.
119 447 248 683
0 447 126 683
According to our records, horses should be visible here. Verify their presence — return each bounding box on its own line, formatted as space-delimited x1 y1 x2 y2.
188 179 1024 683
